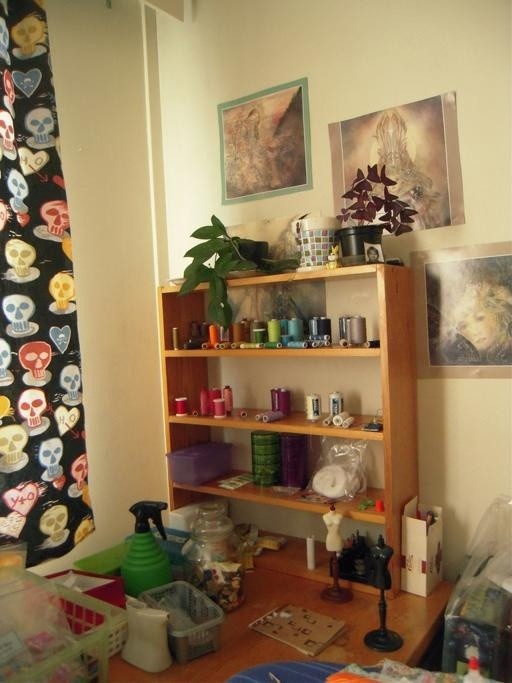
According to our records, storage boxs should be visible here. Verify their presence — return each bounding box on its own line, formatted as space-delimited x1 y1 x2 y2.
39 567 126 636
164 438 236 486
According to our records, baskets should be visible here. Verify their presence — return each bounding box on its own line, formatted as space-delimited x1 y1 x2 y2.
3 565 111 683
139 580 226 665
109 604 128 658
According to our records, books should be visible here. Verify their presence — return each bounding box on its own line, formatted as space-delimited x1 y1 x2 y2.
248 601 348 658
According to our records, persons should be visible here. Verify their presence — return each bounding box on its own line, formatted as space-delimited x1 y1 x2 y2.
371 538 394 591
435 280 511 361
366 246 381 264
322 506 344 552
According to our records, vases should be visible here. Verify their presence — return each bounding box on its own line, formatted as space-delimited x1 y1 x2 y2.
289 214 343 271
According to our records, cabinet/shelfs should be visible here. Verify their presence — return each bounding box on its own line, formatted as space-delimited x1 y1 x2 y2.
156 258 417 601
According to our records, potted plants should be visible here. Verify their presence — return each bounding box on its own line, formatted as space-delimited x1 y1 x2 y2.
333 164 419 267
178 213 300 331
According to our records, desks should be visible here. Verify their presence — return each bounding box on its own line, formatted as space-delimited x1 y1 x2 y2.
99 575 459 680
